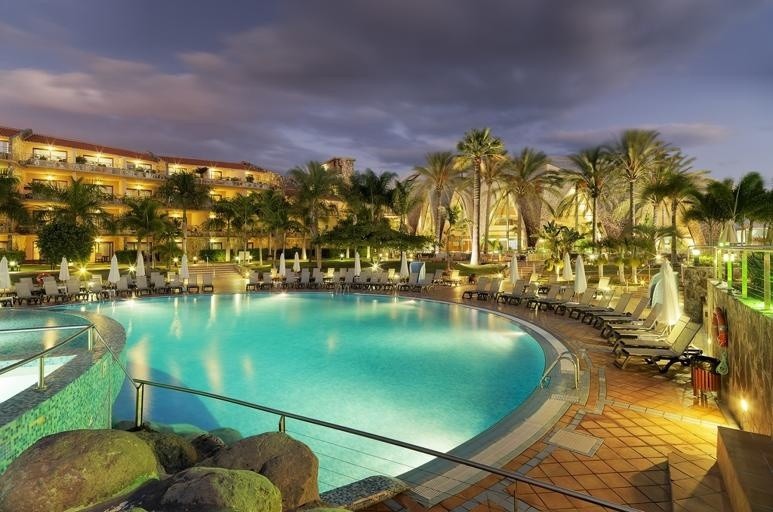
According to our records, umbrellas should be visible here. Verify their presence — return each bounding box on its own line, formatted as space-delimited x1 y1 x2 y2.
354 251 361 277
108 253 120 289
574 254 588 303
135 248 145 279
511 255 518 288
562 251 575 285
60 255 70 285
279 252 286 277
399 252 410 283
178 254 190 283
661 258 682 335
292 251 301 274
0 256 12 298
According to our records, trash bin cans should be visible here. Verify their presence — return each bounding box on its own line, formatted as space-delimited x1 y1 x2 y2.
690 355 721 398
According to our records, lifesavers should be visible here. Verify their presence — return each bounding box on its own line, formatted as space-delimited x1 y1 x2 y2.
713 307 727 347
36 272 48 283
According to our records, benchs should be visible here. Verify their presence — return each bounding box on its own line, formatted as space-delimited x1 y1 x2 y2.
2 268 215 306
462 273 702 373
245 266 462 294
